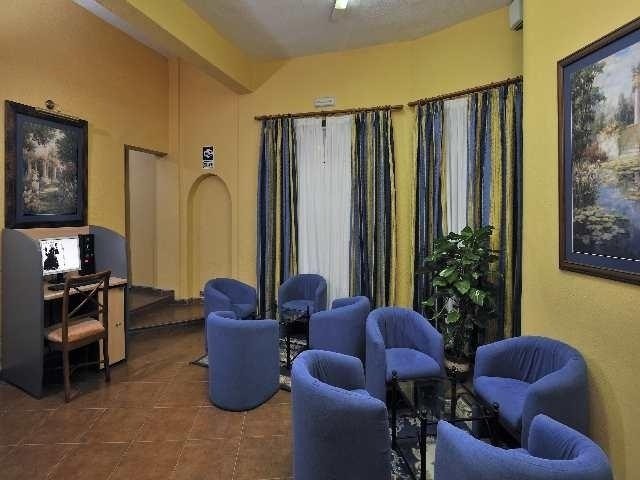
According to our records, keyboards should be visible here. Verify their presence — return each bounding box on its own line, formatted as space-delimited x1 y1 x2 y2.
48 279 97 291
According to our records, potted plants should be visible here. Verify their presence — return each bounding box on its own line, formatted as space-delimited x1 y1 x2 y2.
415 222 508 383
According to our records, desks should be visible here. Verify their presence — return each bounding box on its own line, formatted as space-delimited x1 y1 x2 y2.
43 275 128 372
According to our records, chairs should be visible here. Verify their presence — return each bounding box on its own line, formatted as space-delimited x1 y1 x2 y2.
44 268 112 403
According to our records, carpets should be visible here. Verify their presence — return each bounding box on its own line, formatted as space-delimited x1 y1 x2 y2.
188 336 308 393
387 381 511 480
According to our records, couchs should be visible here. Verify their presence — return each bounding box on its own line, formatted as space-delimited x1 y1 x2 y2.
308 295 371 363
207 310 281 412
290 348 393 480
435 411 614 480
277 271 328 331
471 334 592 450
364 306 447 407
205 275 258 354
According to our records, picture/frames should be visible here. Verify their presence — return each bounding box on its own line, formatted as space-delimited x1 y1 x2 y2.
555 14 640 287
4 97 89 230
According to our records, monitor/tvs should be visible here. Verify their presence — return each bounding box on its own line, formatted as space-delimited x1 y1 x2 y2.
38 237 82 283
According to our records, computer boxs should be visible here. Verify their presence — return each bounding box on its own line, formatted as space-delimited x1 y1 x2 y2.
79 234 95 276
